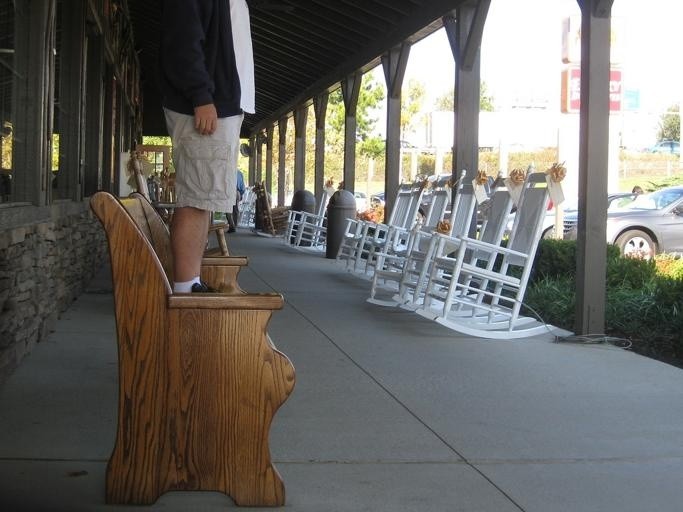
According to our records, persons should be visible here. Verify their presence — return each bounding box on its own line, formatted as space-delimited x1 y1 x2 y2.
224 170 245 233
144 166 170 202
630 186 645 203
136 1 263 297
324 175 336 196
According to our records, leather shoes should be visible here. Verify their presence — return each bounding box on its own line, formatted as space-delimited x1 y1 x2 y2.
192 280 218 292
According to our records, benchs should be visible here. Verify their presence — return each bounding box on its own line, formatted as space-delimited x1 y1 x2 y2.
88 191 295 507
131 151 230 257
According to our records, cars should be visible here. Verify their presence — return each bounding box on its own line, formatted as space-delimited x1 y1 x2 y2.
642 140 680 158
502 187 683 269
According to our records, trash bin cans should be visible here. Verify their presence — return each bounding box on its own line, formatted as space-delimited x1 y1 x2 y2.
289 190 316 246
326 189 356 258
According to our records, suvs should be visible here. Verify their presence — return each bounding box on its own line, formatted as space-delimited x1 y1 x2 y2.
369 172 497 232
